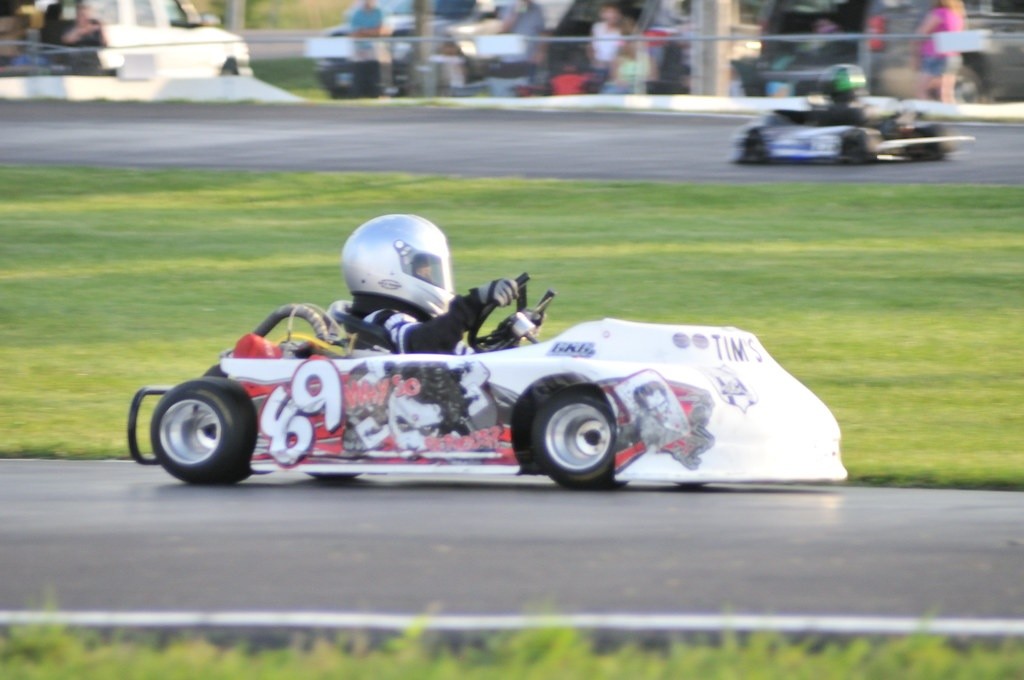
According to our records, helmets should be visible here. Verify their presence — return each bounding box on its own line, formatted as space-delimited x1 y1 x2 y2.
818 62 866 96
343 213 454 319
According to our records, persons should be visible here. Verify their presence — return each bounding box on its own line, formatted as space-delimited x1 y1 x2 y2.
345 0 968 101
339 212 546 356
59 0 108 76
820 62 917 133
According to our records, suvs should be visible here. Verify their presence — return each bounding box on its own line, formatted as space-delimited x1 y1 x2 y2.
28 0 253 77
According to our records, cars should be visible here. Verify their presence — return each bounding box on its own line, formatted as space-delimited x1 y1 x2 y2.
724 1 1024 106
550 1 689 93
309 0 544 101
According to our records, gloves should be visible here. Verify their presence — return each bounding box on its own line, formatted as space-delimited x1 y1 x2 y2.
468 278 520 308
498 310 543 348
